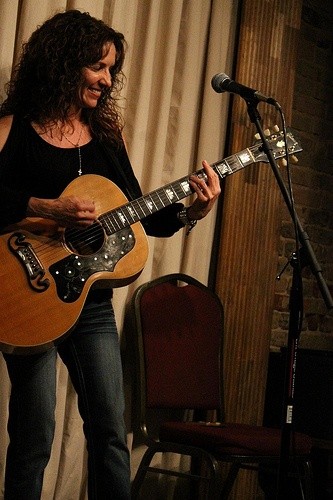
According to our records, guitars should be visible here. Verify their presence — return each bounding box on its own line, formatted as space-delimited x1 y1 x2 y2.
0 123 304 355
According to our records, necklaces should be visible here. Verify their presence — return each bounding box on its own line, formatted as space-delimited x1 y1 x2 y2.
55 122 83 176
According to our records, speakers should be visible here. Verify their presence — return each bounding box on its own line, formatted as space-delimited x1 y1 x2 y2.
257 346 333 500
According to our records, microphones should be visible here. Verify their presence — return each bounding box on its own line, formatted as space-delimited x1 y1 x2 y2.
211 72 279 107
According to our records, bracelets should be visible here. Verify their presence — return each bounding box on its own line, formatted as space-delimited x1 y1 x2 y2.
180 207 197 227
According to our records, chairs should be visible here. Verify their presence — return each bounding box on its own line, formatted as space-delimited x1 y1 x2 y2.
130 274 313 500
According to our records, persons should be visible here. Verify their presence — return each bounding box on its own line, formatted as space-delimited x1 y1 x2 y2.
0 10 221 500
